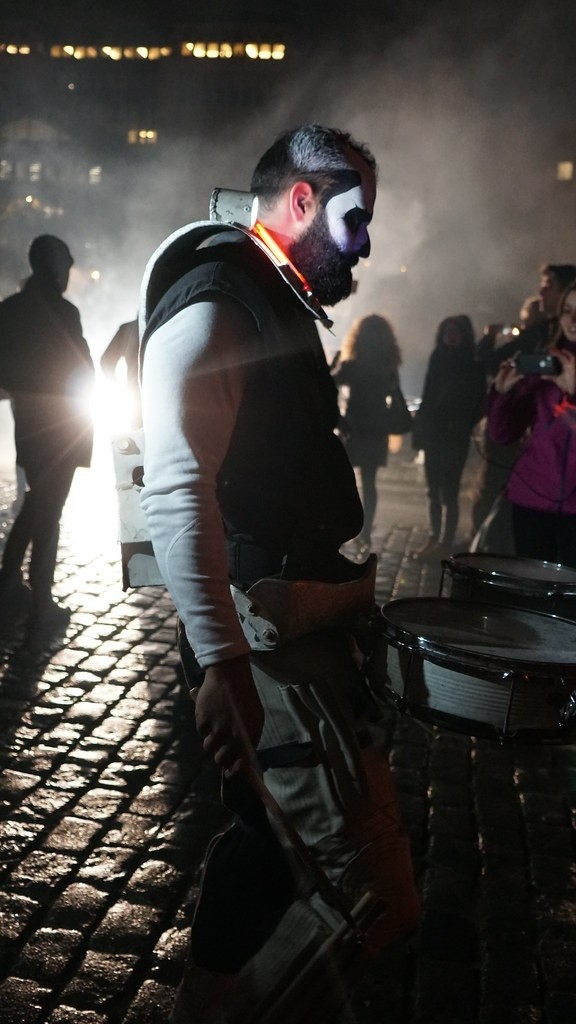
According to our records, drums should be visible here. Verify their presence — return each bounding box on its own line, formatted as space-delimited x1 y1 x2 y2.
372 593 576 753
443 553 576 617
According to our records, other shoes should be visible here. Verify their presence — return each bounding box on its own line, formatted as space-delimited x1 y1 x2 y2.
416 536 439 555
0 570 33 608
27 590 71 625
349 537 371 555
432 542 459 560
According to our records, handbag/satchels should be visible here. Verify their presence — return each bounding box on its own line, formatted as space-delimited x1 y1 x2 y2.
384 366 413 434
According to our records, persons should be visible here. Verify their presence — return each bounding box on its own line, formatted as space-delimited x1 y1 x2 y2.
335 316 412 554
410 315 486 560
1 234 99 620
100 310 143 430
133 124 426 1024
472 265 576 564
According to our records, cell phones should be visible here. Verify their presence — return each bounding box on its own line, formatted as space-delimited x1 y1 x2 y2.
515 353 555 374
